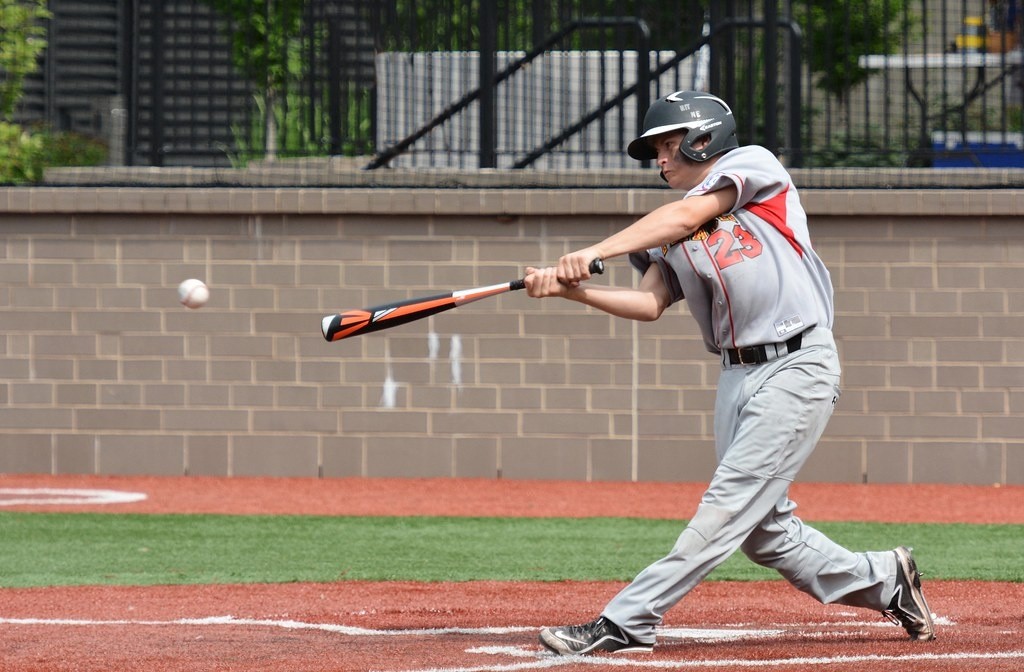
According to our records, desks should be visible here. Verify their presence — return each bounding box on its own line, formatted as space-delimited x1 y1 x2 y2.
855 52 1024 167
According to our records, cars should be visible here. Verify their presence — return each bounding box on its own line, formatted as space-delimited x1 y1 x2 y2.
953 14 1023 56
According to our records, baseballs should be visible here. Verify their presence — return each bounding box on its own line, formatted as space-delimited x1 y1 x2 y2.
177 278 210 309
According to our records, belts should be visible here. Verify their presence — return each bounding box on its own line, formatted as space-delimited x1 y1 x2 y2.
723 332 802 366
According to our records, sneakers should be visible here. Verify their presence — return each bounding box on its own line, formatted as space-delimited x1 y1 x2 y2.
539 616 653 656
882 546 936 641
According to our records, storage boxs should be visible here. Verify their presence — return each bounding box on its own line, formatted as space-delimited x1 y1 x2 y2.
931 130 1023 166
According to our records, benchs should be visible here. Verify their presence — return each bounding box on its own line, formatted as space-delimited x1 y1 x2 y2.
372 51 714 169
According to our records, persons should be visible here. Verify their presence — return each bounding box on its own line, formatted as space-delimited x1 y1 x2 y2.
524 90 935 655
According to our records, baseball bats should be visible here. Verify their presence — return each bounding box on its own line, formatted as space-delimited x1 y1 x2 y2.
320 257 605 343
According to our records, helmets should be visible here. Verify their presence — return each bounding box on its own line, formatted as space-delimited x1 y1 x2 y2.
628 91 739 183
